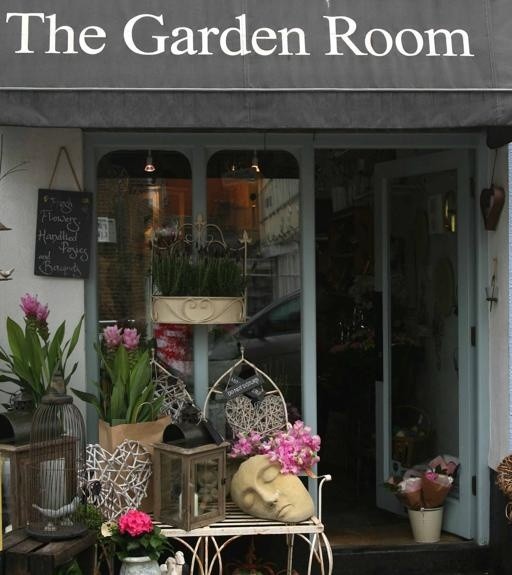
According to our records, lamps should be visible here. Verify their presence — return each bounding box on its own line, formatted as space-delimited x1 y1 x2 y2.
144 150 156 172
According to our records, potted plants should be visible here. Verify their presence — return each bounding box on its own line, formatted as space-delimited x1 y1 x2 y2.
150 213 247 325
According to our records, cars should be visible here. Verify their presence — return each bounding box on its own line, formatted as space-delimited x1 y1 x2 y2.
155 278 302 414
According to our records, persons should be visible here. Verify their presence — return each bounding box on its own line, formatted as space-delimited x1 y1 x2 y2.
196 465 219 512
232 453 315 522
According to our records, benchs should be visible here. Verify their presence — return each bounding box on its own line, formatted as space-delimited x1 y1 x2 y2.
93 475 336 575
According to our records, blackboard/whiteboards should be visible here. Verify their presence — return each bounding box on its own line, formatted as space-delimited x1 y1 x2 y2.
34 188 93 278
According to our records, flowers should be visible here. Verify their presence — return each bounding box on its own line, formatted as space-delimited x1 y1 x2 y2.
227 418 322 474
382 464 456 510
101 508 176 562
0 293 167 423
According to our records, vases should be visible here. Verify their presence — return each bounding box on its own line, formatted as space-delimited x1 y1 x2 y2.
116 550 163 575
